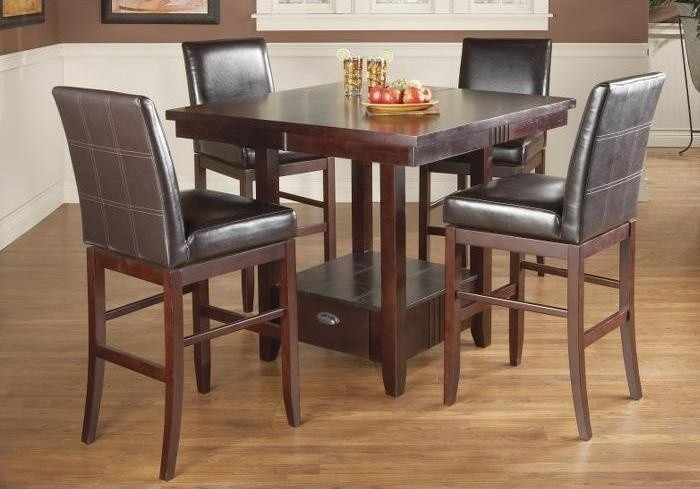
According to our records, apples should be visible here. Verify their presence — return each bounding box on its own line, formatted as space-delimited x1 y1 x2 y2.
370 86 432 104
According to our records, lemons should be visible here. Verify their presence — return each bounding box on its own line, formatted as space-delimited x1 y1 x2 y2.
336 49 350 61
384 51 393 64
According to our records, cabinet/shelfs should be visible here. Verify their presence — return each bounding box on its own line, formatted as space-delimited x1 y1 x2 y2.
272 249 480 360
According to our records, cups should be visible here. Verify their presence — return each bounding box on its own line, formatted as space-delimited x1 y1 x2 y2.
343 56 388 97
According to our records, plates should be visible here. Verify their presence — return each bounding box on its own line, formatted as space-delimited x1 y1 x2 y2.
361 97 434 111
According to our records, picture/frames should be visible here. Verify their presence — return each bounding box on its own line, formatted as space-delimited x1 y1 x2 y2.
101 0 221 25
0 0 46 29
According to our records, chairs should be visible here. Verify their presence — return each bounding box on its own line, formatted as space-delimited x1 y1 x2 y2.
418 37 553 275
182 37 336 313
443 70 666 442
51 86 301 481
